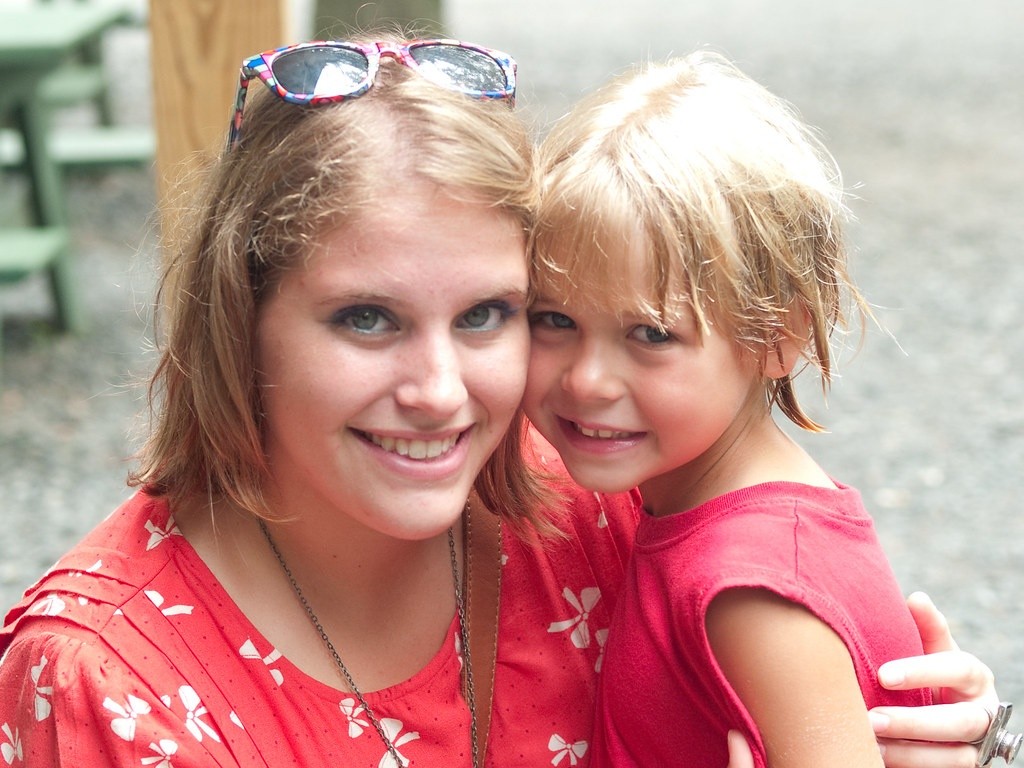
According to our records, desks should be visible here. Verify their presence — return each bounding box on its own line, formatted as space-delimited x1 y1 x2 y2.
1 0 134 332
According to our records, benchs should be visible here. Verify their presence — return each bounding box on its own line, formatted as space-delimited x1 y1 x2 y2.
1 64 157 284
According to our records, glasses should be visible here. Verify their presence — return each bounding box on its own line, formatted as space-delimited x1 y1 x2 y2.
224 37 520 156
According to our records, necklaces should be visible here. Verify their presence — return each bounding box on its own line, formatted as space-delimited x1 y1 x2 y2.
259 515 482 768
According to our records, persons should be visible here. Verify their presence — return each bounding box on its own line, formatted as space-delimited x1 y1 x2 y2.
0 25 1003 768
519 52 949 767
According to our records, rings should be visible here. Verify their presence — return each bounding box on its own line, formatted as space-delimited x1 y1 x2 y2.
970 702 1023 768
